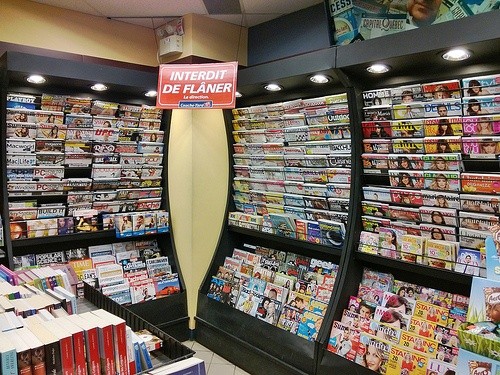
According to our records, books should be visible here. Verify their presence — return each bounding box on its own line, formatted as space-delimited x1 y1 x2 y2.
206 73 500 375
0 90 206 375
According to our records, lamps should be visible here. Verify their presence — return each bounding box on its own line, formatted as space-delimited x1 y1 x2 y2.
158 25 182 57
442 48 474 62
90 83 108 91
264 84 282 91
367 65 391 74
27 75 46 84
309 75 332 84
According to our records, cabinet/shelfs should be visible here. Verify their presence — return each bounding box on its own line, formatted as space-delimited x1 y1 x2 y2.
0 50 190 343
194 46 353 375
316 9 500 375
